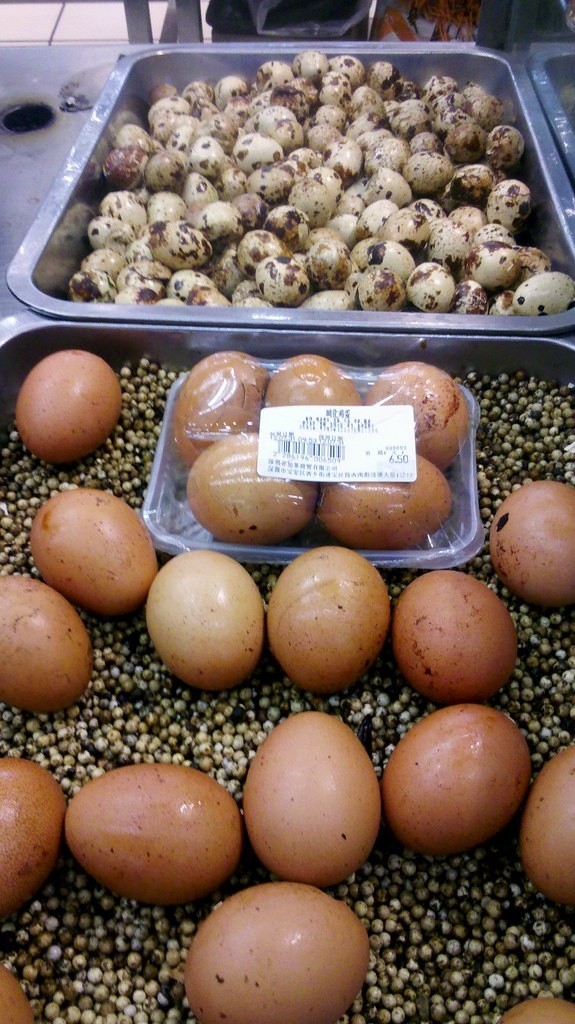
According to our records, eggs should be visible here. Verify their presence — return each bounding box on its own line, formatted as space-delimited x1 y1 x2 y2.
174 350 468 550
16 350 121 463
0 481 575 1024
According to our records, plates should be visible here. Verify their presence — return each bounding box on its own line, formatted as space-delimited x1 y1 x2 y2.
139 360 488 570
